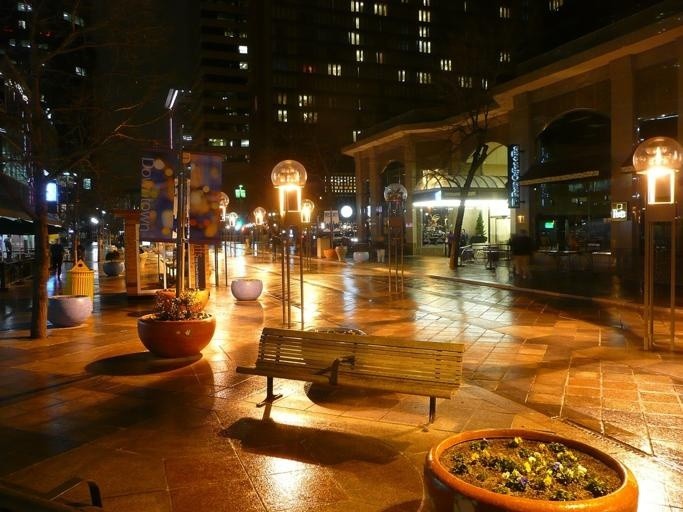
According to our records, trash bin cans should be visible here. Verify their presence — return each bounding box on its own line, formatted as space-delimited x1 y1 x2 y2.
65 259 94 305
317 236 330 259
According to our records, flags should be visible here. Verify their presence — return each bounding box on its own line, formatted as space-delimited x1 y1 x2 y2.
136 146 179 244
186 151 229 245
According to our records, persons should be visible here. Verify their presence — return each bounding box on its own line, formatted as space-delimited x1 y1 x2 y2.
446 230 454 258
511 230 533 281
71 240 85 265
374 234 386 262
3 235 13 262
49 239 66 281
458 228 469 257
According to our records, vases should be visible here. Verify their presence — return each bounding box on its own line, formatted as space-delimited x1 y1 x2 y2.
422 427 640 510
230 277 262 302
322 244 386 263
102 261 124 276
136 310 215 358
46 294 93 328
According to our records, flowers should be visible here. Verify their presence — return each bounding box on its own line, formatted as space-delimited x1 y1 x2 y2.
449 433 611 501
150 286 206 319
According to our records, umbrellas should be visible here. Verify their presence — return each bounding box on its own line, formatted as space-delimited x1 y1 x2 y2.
12 219 34 261
30 221 64 235
0 217 35 261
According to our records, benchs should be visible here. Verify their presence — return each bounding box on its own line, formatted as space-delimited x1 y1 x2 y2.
235 327 464 432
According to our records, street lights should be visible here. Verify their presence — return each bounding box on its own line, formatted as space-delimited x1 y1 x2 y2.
629 135 682 354
211 160 409 337
